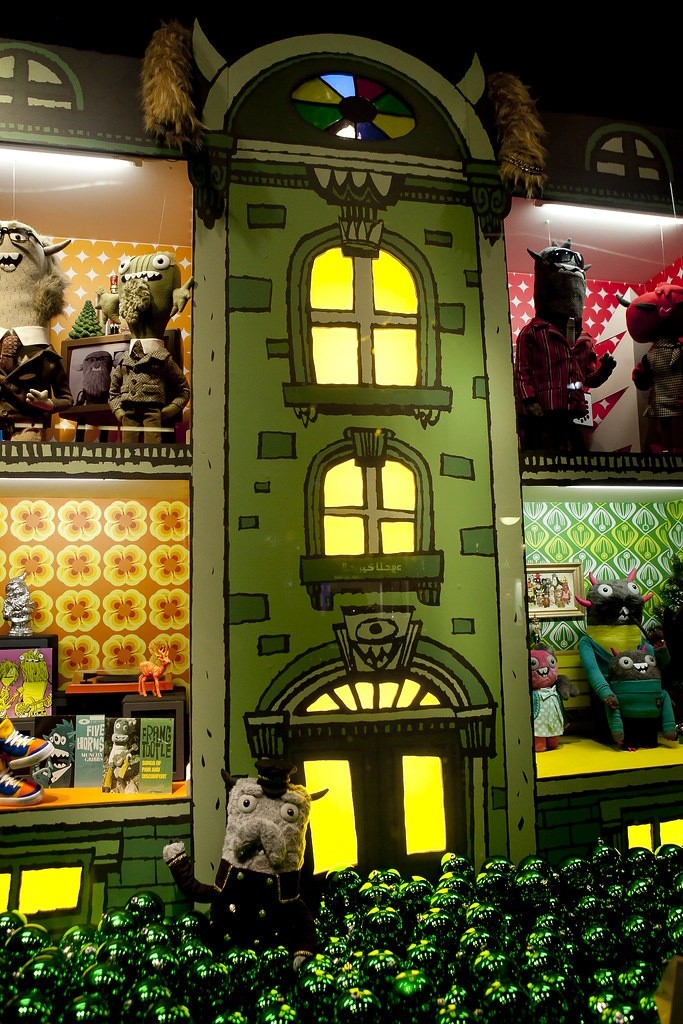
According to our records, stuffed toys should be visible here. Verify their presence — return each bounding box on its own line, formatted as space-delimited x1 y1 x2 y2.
0 220 74 442
514 239 618 452
101 253 194 444
161 760 329 953
615 284 683 457
530 567 679 753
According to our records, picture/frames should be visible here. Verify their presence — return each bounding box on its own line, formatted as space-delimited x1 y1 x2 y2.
57 329 181 427
523 561 586 619
